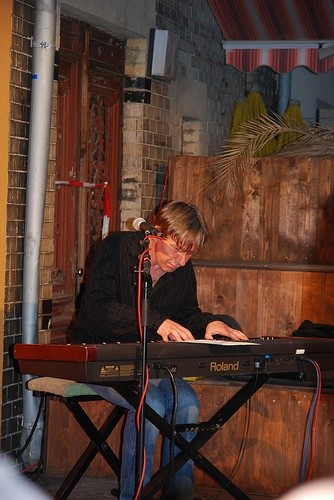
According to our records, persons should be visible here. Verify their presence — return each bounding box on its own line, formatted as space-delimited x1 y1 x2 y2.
70 200 250 500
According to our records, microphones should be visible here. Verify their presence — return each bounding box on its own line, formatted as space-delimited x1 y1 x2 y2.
132 217 168 239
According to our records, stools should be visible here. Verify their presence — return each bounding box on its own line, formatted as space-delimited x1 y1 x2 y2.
27 377 139 500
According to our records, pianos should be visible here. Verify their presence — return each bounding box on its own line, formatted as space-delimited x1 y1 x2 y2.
13 336 334 500
